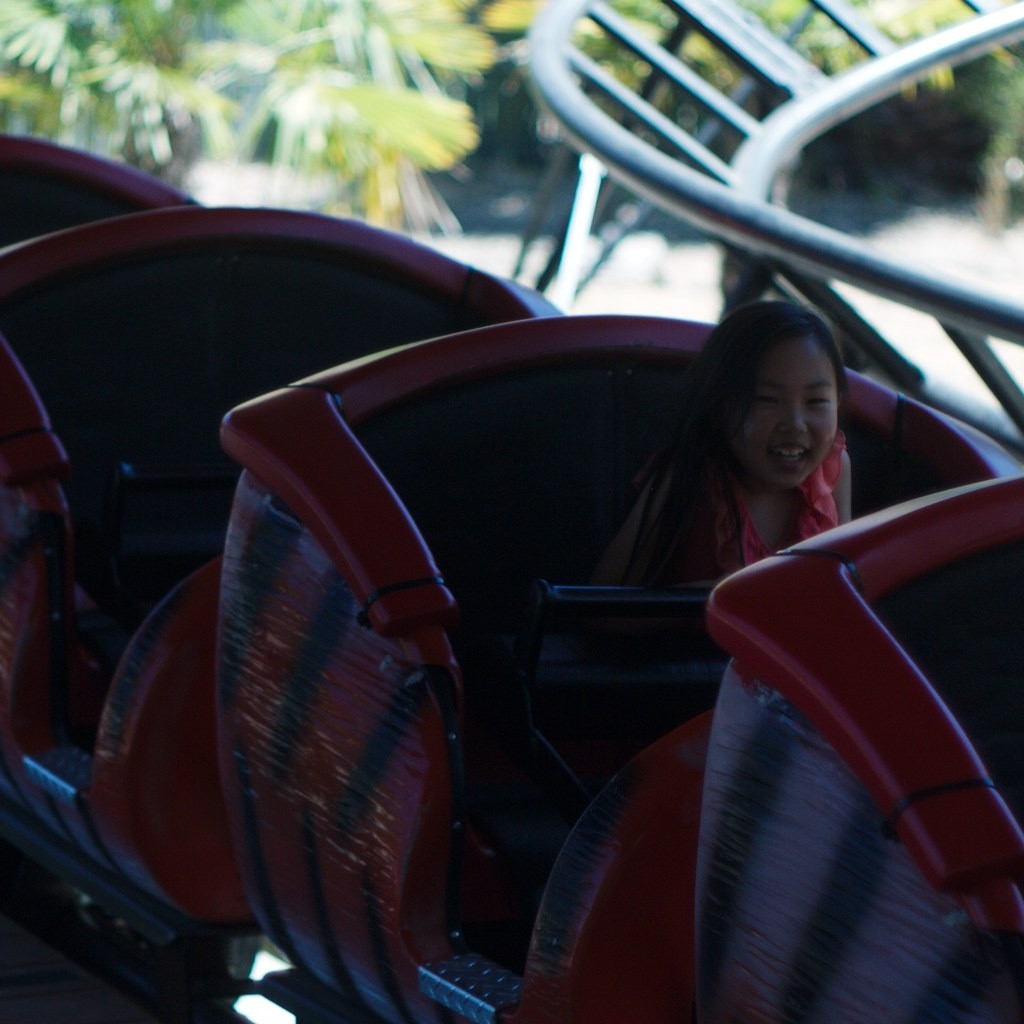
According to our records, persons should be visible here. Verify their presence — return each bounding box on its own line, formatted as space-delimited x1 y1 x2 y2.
593 299 852 589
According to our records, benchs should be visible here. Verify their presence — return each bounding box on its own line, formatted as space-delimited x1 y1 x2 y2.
0 135 1024 1024
